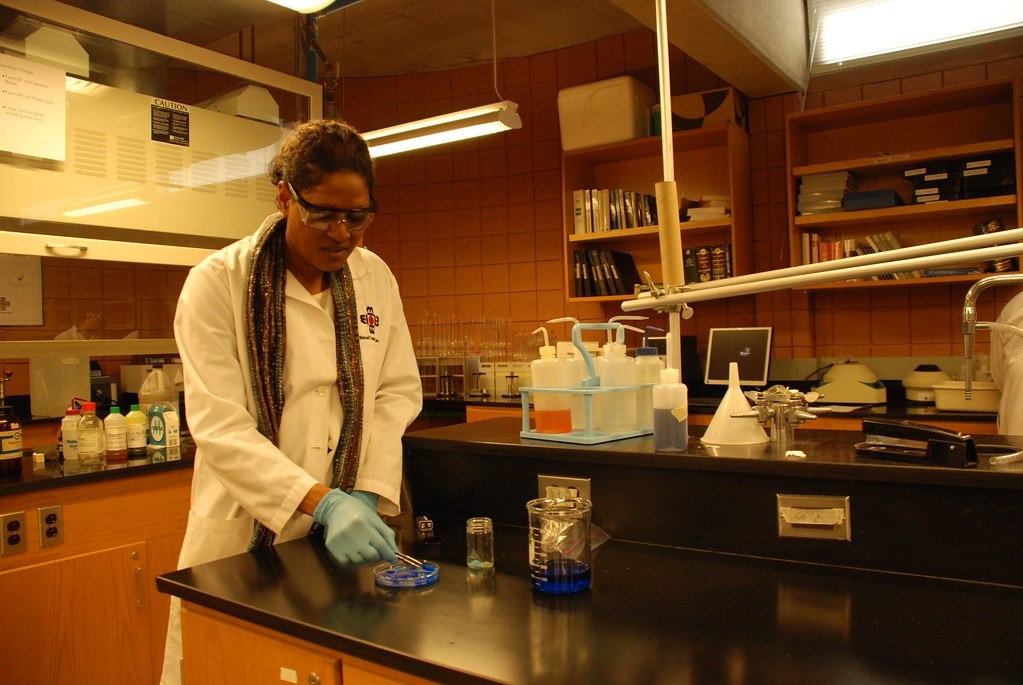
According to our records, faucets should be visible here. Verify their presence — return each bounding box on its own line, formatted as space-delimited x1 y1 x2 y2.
962 320 976 402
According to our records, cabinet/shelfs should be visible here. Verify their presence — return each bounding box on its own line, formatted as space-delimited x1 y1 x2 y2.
562 124 751 299
784 75 1023 283
0 466 198 684
179 597 429 685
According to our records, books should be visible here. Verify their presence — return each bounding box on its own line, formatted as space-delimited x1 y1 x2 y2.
797 159 1015 216
682 244 733 286
572 189 732 234
802 219 1016 282
573 249 644 297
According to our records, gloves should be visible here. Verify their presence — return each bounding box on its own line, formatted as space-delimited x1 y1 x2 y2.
311 487 401 571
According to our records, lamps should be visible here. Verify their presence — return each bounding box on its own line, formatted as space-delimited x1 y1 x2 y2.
339 0 523 158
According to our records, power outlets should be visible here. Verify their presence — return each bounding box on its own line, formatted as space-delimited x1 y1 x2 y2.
37 504 63 547
0 510 27 557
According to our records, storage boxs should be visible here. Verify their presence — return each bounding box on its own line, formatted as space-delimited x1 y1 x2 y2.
650 85 752 136
558 74 655 150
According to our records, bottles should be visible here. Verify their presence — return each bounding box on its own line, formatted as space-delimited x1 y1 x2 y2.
104 407 128 461
60 409 82 460
652 368 688 452
124 404 147 460
76 401 103 466
0 406 24 477
138 363 180 430
466 518 495 570
531 344 666 434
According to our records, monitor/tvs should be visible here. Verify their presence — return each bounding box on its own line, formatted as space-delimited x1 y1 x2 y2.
704 327 772 387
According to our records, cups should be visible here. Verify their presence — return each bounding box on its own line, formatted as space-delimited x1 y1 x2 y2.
526 496 592 595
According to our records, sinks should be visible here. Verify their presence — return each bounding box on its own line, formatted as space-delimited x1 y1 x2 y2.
974 444 1021 455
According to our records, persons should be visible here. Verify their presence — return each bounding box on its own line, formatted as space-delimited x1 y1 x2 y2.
990 290 1023 436
158 121 423 685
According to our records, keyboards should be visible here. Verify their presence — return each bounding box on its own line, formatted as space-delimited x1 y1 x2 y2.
688 397 756 413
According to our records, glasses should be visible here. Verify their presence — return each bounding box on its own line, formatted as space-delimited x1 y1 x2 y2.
284 176 378 232
